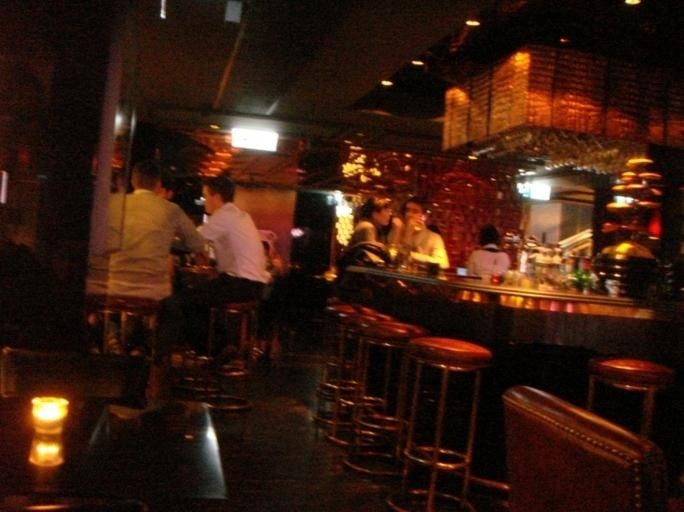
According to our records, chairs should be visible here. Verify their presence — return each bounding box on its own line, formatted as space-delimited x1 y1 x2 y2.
500 384 667 512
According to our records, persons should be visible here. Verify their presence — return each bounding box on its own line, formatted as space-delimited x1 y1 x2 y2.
397 194 450 279
336 195 402 312
157 173 180 201
462 223 511 279
102 159 206 310
175 176 271 361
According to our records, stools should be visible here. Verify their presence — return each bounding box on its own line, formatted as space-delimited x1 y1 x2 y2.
343 320 428 479
586 357 674 438
312 302 377 426
105 296 159 359
386 336 493 512
322 313 394 447
198 301 259 412
